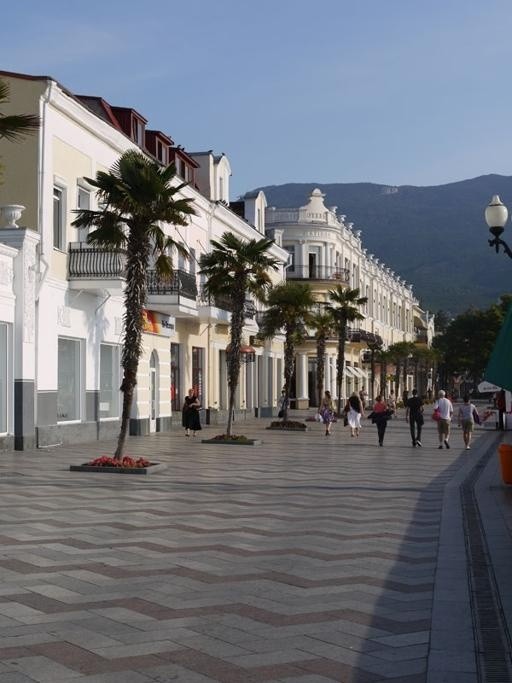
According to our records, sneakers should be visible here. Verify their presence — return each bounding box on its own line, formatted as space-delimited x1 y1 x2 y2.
326 430 471 450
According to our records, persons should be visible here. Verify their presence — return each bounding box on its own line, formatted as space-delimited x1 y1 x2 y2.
181 389 201 436
317 383 483 451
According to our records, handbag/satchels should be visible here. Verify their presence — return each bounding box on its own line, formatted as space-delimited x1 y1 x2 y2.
433 410 440 421
416 412 423 426
345 404 350 411
474 415 482 425
278 410 285 418
344 415 348 426
